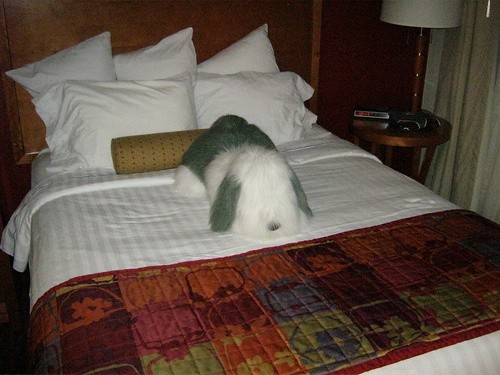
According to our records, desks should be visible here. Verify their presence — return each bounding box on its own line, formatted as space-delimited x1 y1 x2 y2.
352 115 452 186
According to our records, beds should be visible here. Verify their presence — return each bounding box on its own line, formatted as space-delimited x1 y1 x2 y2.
30 124 500 375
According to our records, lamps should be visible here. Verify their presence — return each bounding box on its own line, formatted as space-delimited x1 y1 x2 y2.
380 0 465 130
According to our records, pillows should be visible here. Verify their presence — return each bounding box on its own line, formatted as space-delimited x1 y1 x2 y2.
5 32 116 96
191 71 319 147
30 71 198 174
112 27 198 80
110 129 209 175
198 24 280 75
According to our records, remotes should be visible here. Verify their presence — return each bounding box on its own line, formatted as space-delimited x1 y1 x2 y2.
354 110 390 119
